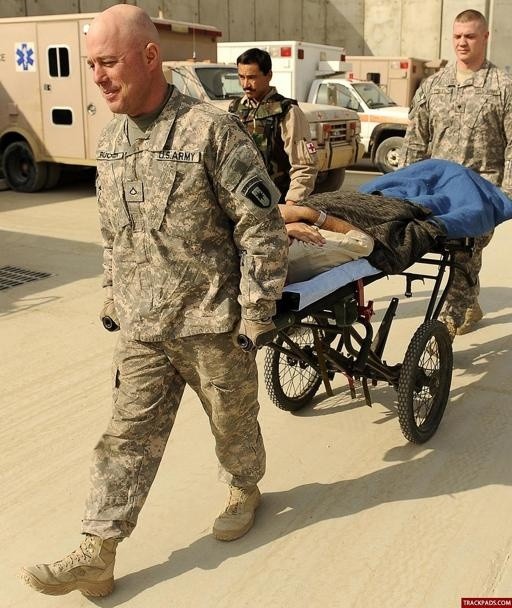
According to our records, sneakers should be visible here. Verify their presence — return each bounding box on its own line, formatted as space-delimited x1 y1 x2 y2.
426 297 483 359
19 536 118 597
213 474 261 541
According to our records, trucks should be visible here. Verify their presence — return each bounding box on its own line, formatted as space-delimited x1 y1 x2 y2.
219 40 421 173
343 56 449 109
0 13 361 193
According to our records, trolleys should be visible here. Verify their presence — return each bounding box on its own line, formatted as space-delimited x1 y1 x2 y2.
101 232 477 445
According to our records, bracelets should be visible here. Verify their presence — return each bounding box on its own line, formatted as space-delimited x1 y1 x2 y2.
314 210 327 227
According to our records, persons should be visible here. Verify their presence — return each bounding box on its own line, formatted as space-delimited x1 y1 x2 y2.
19 4 289 597
228 48 319 205
399 9 512 357
277 191 450 284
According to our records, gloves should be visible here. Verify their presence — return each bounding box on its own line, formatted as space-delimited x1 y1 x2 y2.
99 296 120 332
231 317 278 352
